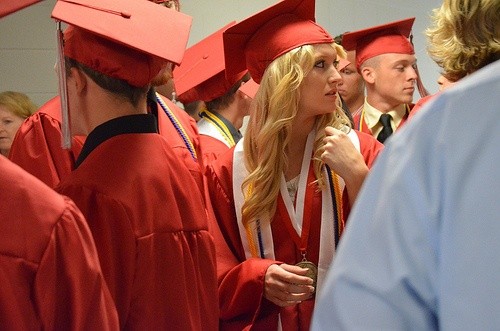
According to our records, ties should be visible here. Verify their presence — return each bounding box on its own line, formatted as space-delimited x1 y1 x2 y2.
377 114 393 143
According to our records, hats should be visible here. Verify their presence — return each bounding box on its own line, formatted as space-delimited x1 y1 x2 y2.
342 18 426 97
173 21 237 102
223 0 334 84
50 0 192 148
